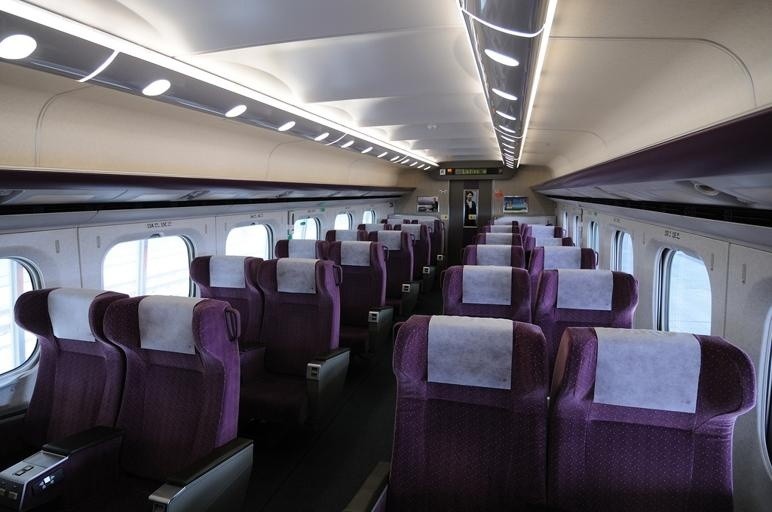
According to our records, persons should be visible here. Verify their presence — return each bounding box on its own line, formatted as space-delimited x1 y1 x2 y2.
465 191 476 226
